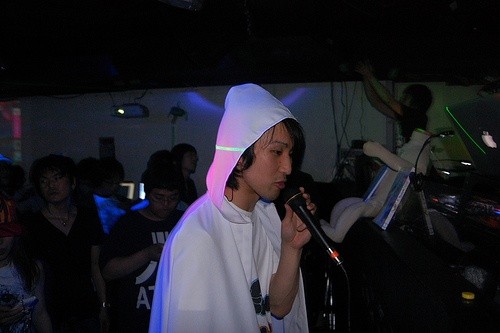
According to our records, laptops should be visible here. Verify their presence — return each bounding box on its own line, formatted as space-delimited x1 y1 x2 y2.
444 93 500 175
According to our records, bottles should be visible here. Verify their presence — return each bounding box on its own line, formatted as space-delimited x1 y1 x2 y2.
459 291 477 327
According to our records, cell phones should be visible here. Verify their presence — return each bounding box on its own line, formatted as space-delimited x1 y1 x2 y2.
10 296 36 311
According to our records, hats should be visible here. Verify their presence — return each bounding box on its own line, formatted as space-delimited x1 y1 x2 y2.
0 196 23 237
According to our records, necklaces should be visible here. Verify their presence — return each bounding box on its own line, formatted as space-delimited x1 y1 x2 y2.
46 205 73 226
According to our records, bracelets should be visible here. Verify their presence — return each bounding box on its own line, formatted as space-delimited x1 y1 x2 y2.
101 302 110 311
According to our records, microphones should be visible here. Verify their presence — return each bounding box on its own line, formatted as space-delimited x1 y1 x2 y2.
285 190 344 265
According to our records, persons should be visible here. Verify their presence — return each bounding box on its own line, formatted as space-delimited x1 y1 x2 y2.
0 144 200 333
148 83 317 333
355 61 434 144
